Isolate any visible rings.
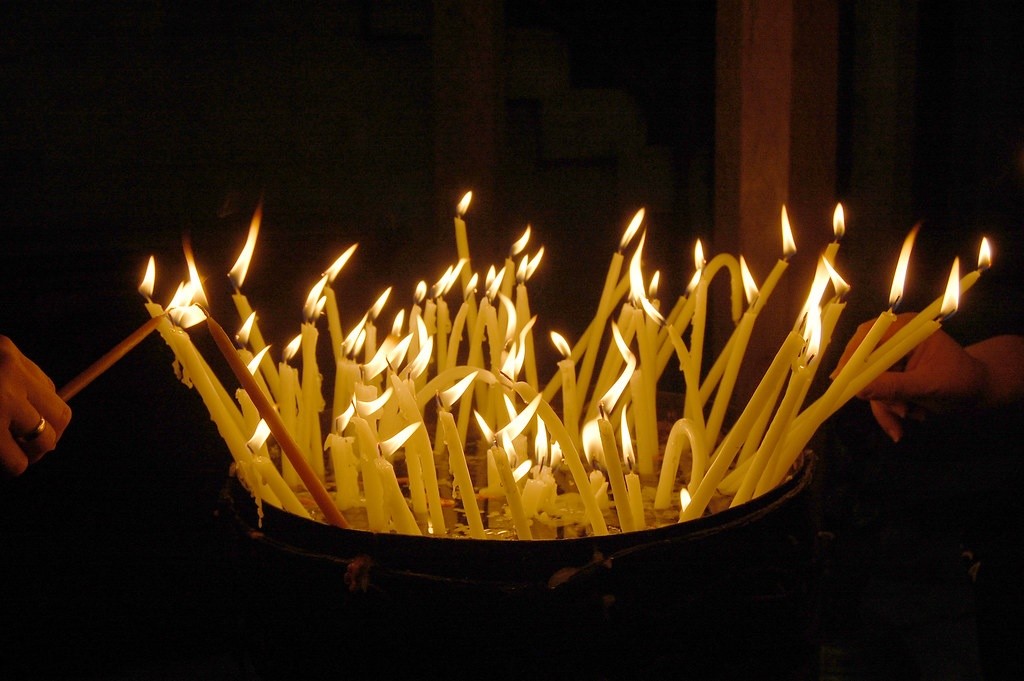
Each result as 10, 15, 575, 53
18, 415, 46, 444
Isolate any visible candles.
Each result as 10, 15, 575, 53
137, 192, 994, 542
57, 274, 210, 404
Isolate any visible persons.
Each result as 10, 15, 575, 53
0, 334, 73, 477
827, 310, 1024, 444
